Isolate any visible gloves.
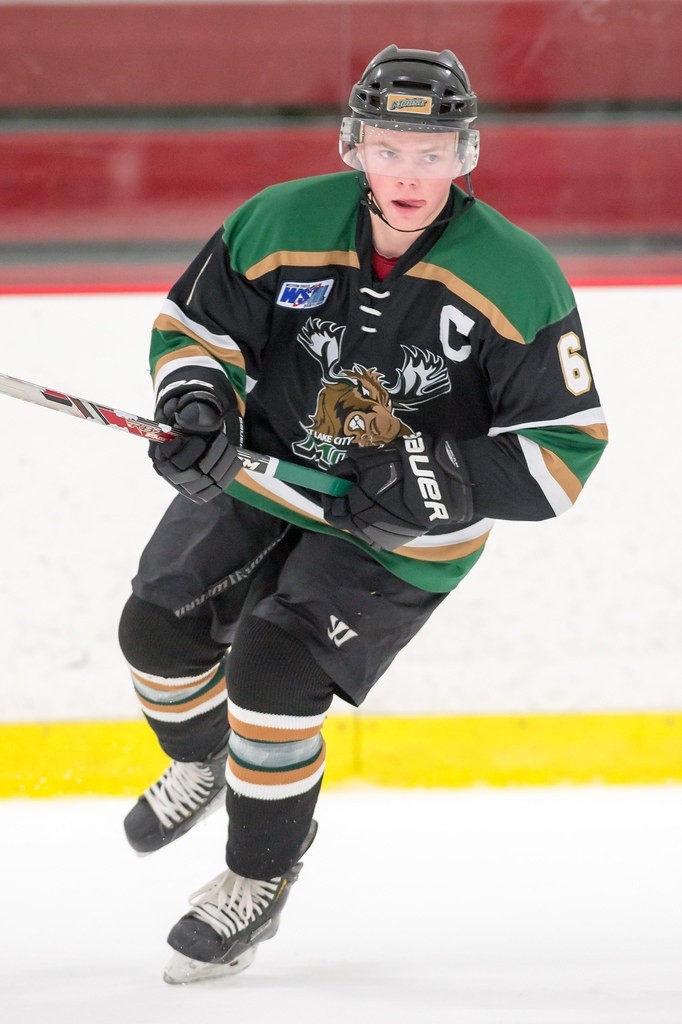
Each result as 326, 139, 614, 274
146, 389, 243, 506
319, 432, 474, 530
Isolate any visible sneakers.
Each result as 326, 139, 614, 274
123, 727, 231, 857
163, 819, 319, 985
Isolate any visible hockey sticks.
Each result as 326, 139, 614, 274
0, 369, 356, 498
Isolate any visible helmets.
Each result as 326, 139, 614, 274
348, 43, 478, 160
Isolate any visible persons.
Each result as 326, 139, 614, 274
115, 43, 611, 986
